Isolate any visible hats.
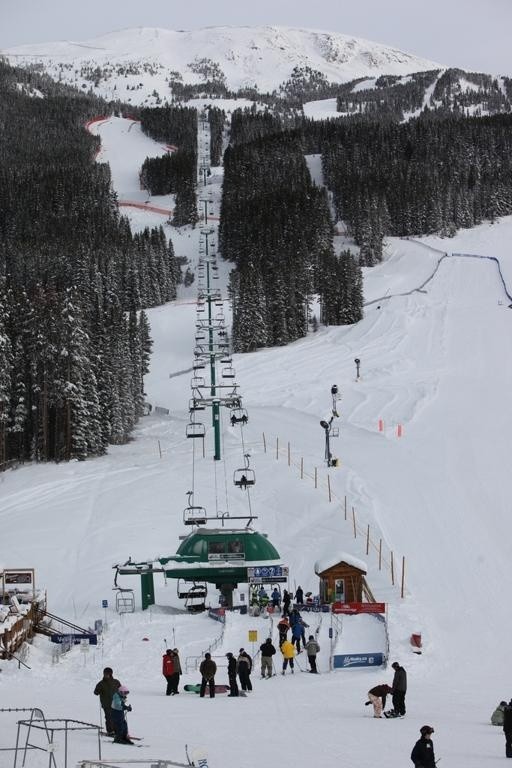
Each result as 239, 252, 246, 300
420, 726, 433, 736
118, 687, 129, 695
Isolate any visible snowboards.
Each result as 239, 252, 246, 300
384, 710, 402, 717
184, 685, 226, 693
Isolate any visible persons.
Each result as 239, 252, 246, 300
501, 697, 512, 758
367, 683, 391, 719
159, 579, 322, 700
111, 685, 136, 745
491, 699, 507, 726
231, 414, 237, 426
410, 724, 437, 768
93, 666, 123, 733
242, 413, 248, 425
389, 661, 408, 718
238, 475, 248, 489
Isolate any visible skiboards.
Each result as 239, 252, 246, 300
260, 665, 321, 680
98, 730, 151, 749
